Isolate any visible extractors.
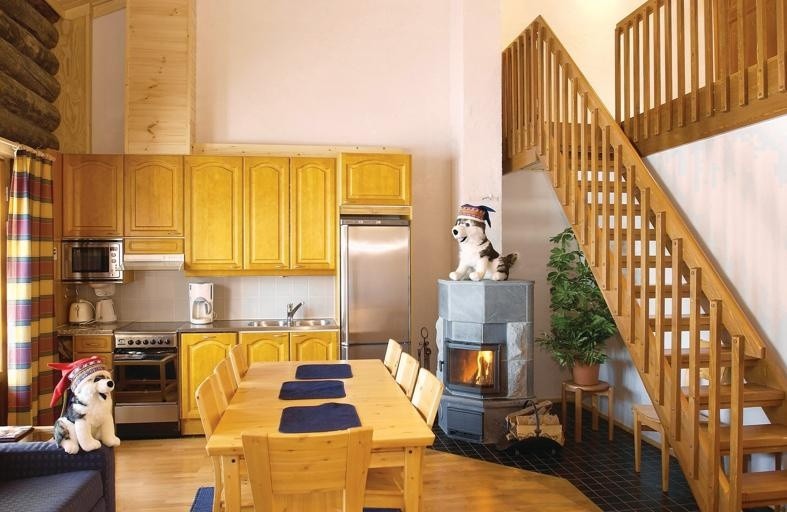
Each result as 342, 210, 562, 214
122, 254, 184, 273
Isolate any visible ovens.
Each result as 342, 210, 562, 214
112, 348, 183, 441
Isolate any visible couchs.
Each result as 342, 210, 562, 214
1, 441, 115, 512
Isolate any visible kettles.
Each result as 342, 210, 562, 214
95, 298, 117, 323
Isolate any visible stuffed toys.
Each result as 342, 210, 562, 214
47, 355, 121, 455
448, 204, 518, 281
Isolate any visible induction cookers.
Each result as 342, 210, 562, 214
112, 319, 186, 348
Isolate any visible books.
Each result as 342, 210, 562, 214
0, 425, 33, 442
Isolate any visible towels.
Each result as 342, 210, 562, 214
296, 364, 353, 380
279, 403, 362, 432
279, 381, 346, 400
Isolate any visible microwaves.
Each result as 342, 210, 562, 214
60, 239, 124, 285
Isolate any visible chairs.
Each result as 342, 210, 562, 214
394, 351, 420, 400
241, 426, 374, 512
631, 339, 730, 491
228, 342, 249, 384
195, 375, 249, 512
364, 367, 445, 511
214, 359, 235, 405
384, 338, 403, 377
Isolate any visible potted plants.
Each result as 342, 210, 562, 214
532, 227, 618, 385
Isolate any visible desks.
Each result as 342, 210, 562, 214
0, 426, 35, 443
204, 358, 435, 512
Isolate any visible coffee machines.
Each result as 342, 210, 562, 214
188, 281, 214, 325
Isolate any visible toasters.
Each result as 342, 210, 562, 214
68, 300, 94, 324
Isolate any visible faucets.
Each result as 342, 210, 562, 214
285, 300, 305, 320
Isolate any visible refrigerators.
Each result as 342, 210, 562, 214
339, 217, 411, 362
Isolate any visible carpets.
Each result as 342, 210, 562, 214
190, 487, 403, 512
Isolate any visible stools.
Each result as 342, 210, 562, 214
561, 379, 615, 443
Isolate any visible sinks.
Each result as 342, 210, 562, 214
246, 318, 331, 329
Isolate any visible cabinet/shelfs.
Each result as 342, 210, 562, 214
72, 334, 113, 372
340, 151, 412, 206
184, 156, 243, 271
62, 153, 184, 255
179, 332, 238, 435
239, 330, 341, 367
244, 156, 337, 271
